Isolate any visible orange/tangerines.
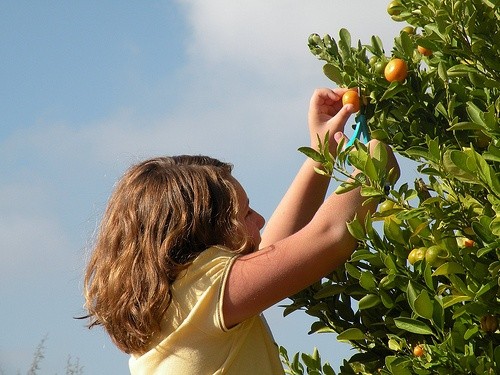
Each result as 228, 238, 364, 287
380, 198, 475, 266
414, 345, 423, 358
341, 1, 433, 114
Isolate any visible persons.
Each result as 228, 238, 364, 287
74, 86, 402, 375
